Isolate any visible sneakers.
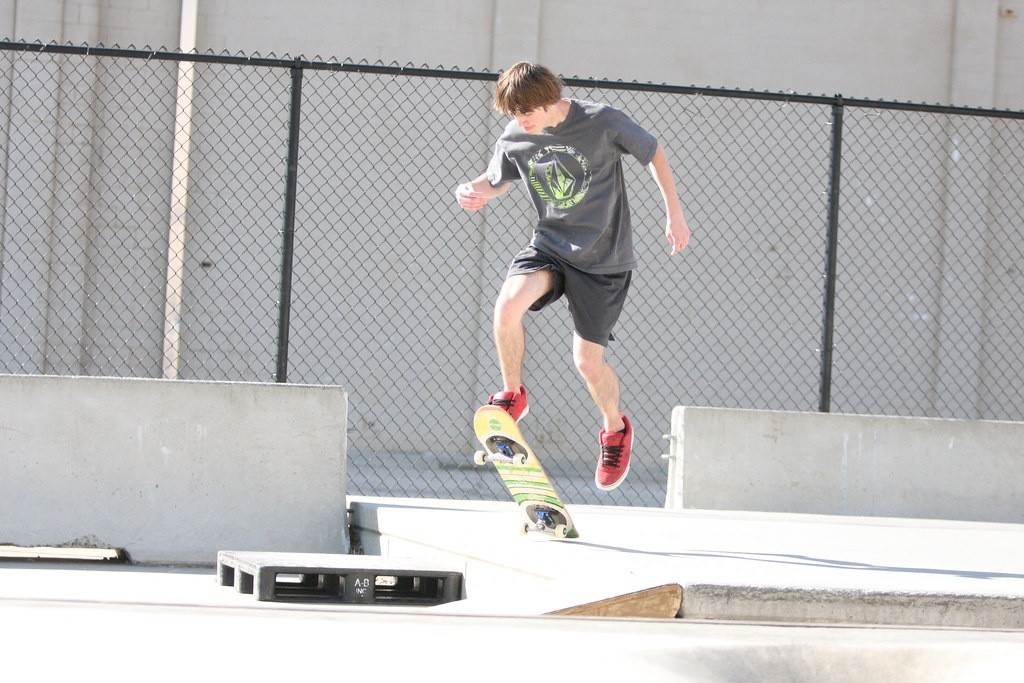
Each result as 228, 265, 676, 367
595, 412, 634, 492
487, 384, 529, 424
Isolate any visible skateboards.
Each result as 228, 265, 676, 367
473, 405, 579, 538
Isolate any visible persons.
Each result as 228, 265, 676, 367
455, 61, 689, 491
